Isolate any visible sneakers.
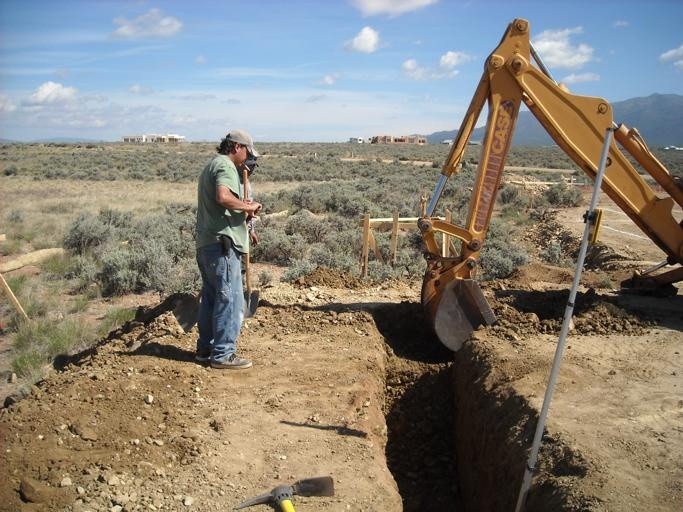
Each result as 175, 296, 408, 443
194, 354, 211, 361
210, 354, 253, 370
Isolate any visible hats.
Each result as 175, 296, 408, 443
226, 129, 261, 168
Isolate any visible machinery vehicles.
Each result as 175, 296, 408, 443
410, 20, 682, 354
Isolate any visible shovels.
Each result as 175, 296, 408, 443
242, 169, 259, 319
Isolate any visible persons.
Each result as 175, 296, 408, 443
196, 128, 262, 369
235, 147, 259, 318
